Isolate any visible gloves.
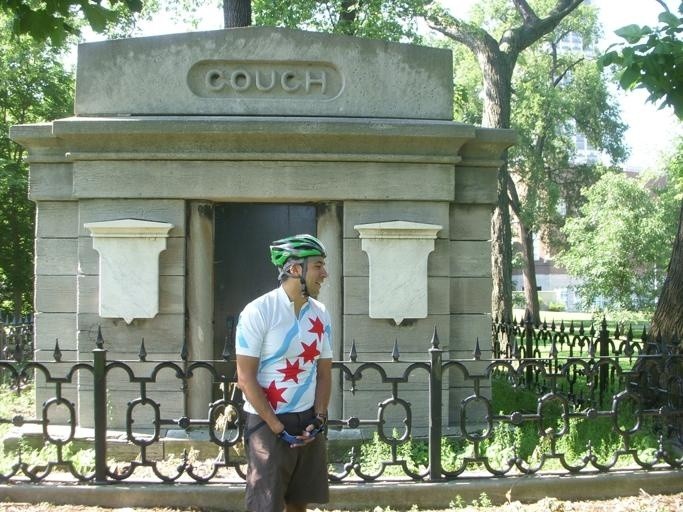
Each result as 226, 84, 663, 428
276, 418, 324, 445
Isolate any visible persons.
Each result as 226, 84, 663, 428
235, 235, 332, 511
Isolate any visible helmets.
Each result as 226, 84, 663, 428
269, 234, 326, 266
